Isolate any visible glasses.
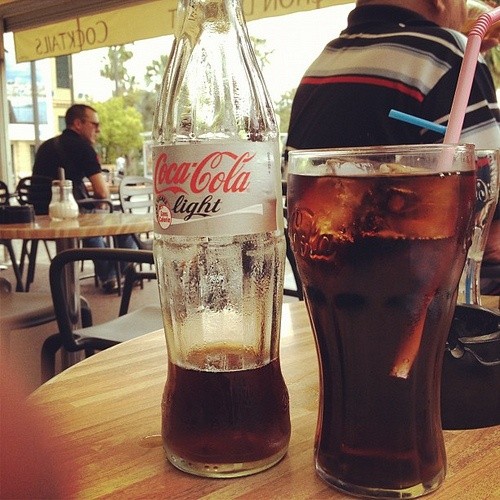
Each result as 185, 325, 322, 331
89, 121, 99, 128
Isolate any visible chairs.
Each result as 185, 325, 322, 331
0, 175, 303, 385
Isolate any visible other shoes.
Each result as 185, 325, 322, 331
101, 275, 126, 292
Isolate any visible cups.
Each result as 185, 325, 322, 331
287, 143, 474, 500
455, 148, 498, 305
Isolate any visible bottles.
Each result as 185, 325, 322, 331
48, 180, 80, 221
149, 0, 291, 479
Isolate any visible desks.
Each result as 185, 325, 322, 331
27, 296, 500, 500
0, 212, 153, 371
85, 181, 121, 211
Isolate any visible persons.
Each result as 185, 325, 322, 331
28, 104, 143, 295
283, 1, 500, 298
